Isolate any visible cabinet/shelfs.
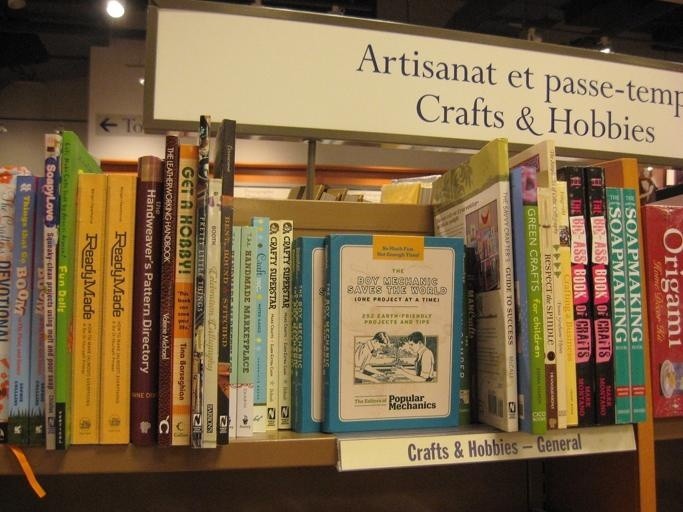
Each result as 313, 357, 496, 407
0, 157, 682, 512
